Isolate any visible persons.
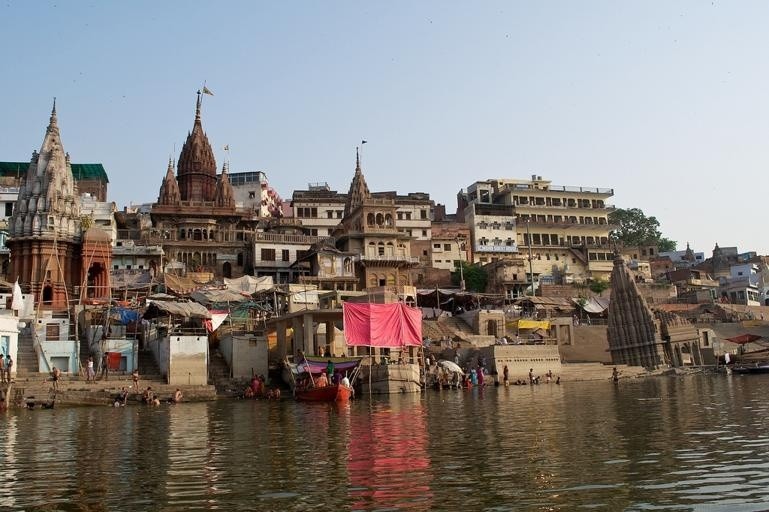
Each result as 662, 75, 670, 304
721, 295, 730, 304
5, 355, 13, 382
733, 309, 764, 321
0, 397, 5, 411
609, 367, 622, 382
52, 366, 59, 390
0, 354, 5, 382
422, 335, 560, 389
587, 313, 591, 325
453, 300, 477, 315
86, 357, 96, 381
573, 313, 579, 326
523, 306, 528, 318
294, 344, 351, 387
115, 366, 183, 406
243, 374, 281, 400
101, 351, 111, 380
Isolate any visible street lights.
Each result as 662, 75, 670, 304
523, 216, 535, 297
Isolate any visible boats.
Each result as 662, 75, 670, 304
294, 382, 352, 402
733, 363, 769, 374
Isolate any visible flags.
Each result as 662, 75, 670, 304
223, 144, 229, 150
202, 85, 214, 97
361, 140, 368, 145
